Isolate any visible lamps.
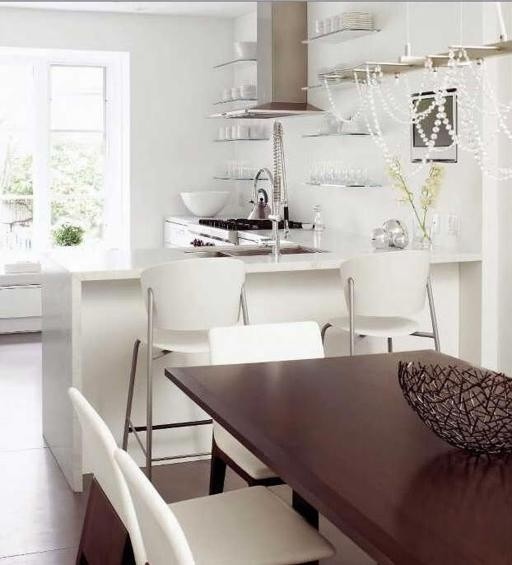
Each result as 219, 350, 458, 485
322, 0, 512, 181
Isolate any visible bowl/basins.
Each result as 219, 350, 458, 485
301, 223, 314, 231
232, 86, 256, 100
235, 41, 258, 59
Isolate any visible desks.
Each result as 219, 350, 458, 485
164, 346, 511, 565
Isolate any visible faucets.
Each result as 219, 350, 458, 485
250, 166, 283, 264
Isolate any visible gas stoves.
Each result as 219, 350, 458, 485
187, 219, 315, 249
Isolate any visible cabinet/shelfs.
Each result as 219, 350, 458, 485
211, 58, 270, 183
302, 28, 390, 191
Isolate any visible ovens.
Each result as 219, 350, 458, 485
410, 92, 458, 163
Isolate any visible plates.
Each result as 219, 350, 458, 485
340, 11, 374, 30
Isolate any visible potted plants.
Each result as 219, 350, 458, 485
50, 221, 86, 256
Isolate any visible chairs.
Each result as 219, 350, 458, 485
209, 320, 326, 533
67, 385, 338, 565
124, 257, 248, 482
321, 252, 439, 356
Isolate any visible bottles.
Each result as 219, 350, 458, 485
311, 203, 321, 232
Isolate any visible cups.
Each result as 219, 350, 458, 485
316, 16, 341, 35
218, 126, 249, 139
222, 161, 260, 180
309, 160, 368, 185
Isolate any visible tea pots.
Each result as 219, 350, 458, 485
248, 187, 272, 220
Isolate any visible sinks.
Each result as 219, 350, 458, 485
227, 243, 323, 258
185, 247, 233, 258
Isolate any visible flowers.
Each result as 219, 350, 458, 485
388, 157, 445, 240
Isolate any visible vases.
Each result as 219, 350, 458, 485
414, 212, 437, 252
